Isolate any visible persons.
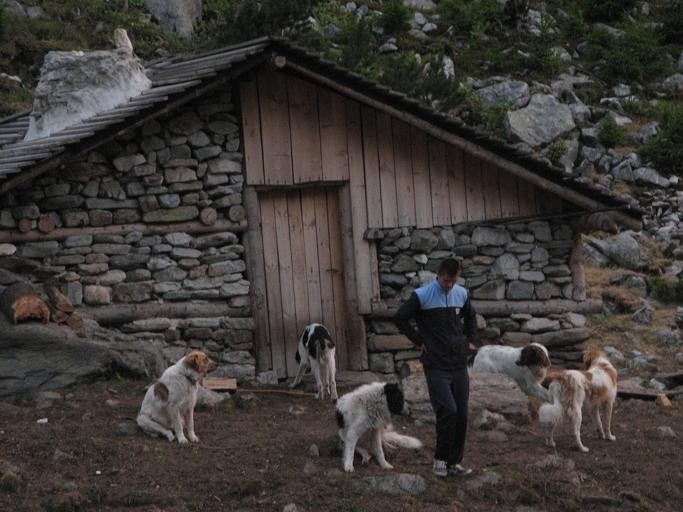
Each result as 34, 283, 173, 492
393, 258, 479, 477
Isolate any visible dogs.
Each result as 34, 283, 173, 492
137, 350, 220, 446
461, 342, 553, 406
290, 322, 337, 403
537, 350, 618, 453
335, 381, 422, 473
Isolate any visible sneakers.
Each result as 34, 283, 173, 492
432, 457, 473, 477
